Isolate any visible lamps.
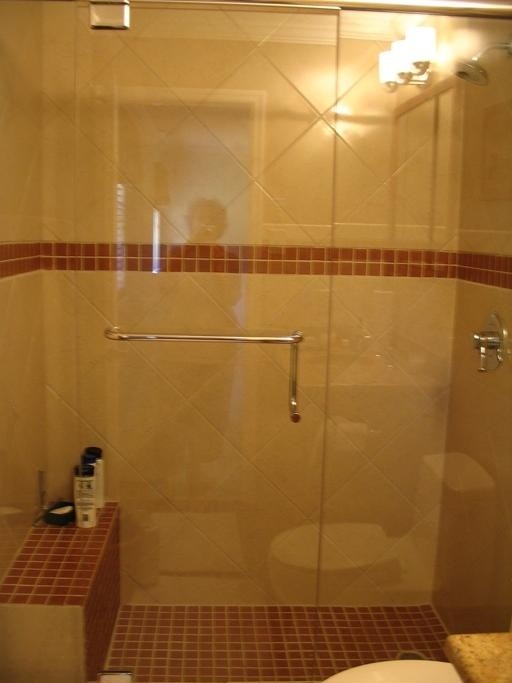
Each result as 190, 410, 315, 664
379, 25, 437, 93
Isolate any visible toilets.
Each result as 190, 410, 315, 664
321, 659, 462, 682
269, 451, 496, 610
